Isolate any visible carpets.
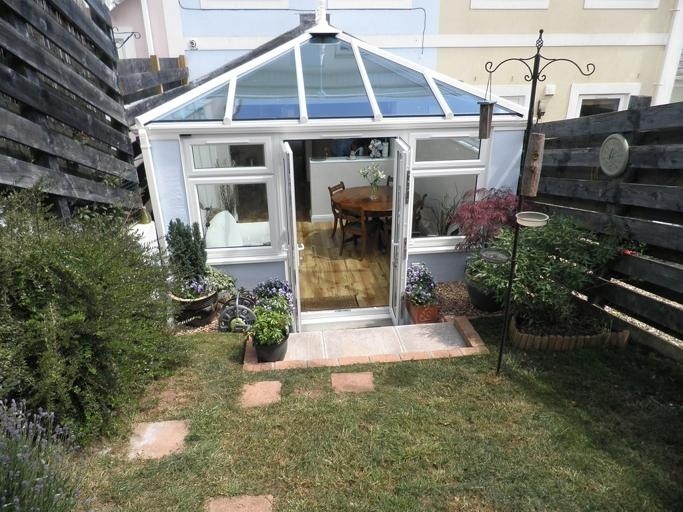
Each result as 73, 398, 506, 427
300, 293, 360, 311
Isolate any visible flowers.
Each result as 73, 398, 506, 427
170, 272, 218, 299
402, 263, 444, 308
359, 164, 387, 195
251, 278, 296, 326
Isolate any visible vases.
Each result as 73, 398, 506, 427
168, 291, 219, 327
367, 183, 382, 201
406, 300, 443, 324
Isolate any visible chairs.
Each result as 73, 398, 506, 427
328, 176, 427, 261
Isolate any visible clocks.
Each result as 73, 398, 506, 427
598, 133, 629, 178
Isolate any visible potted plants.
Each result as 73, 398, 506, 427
240, 305, 294, 362
